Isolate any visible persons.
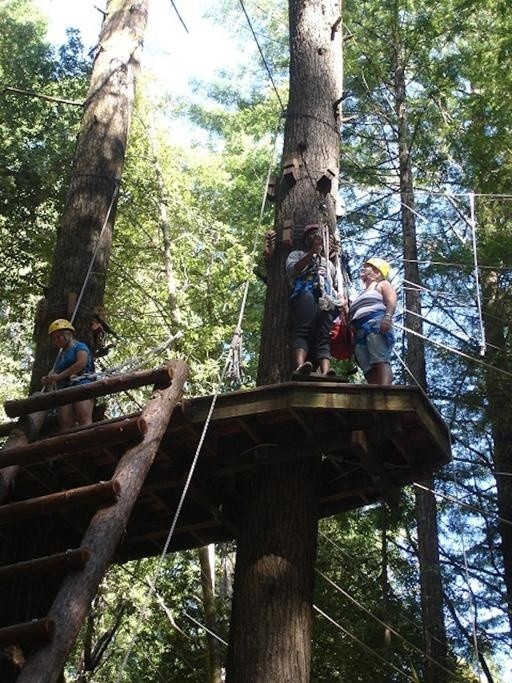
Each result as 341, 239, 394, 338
286, 223, 339, 376
39, 317, 96, 430
346, 257, 398, 383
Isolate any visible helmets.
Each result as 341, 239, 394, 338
48, 319, 75, 335
367, 258, 389, 279
303, 224, 319, 233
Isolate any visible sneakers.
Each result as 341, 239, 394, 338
296, 361, 313, 372
326, 368, 337, 376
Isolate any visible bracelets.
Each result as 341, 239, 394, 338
383, 313, 393, 320
